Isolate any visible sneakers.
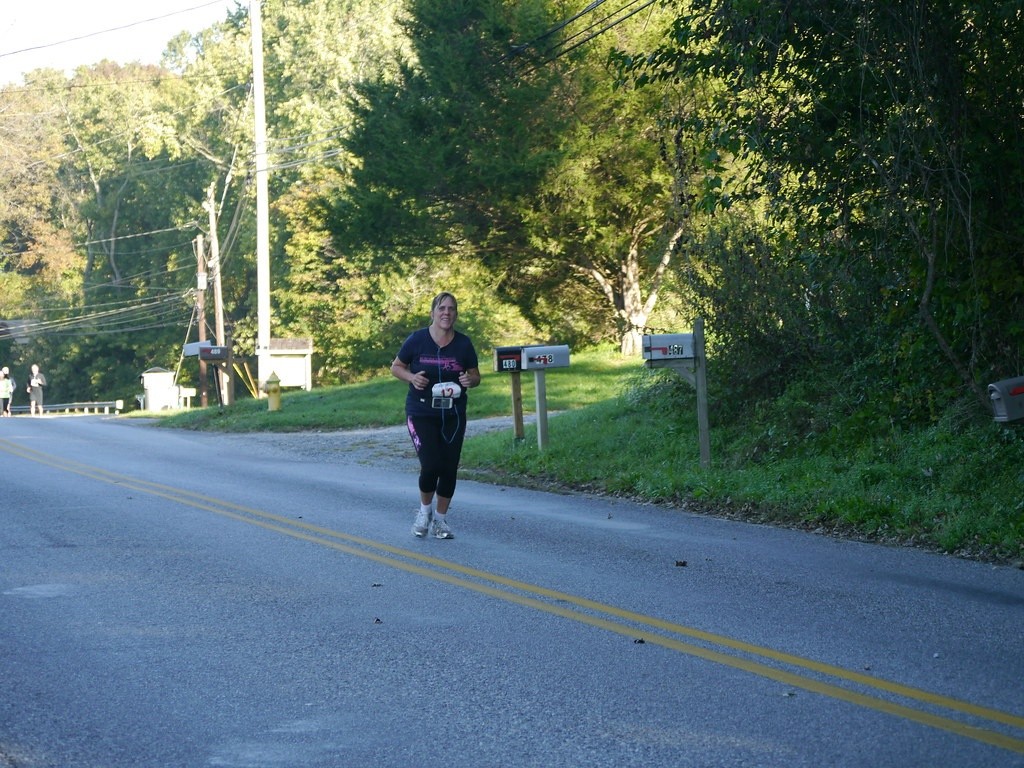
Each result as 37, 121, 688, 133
430, 517, 454, 539
411, 510, 433, 538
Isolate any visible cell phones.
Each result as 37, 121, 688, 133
432, 398, 453, 409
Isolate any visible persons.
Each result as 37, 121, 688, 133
25, 363, 47, 417
0, 365, 17, 417
390, 292, 481, 542
